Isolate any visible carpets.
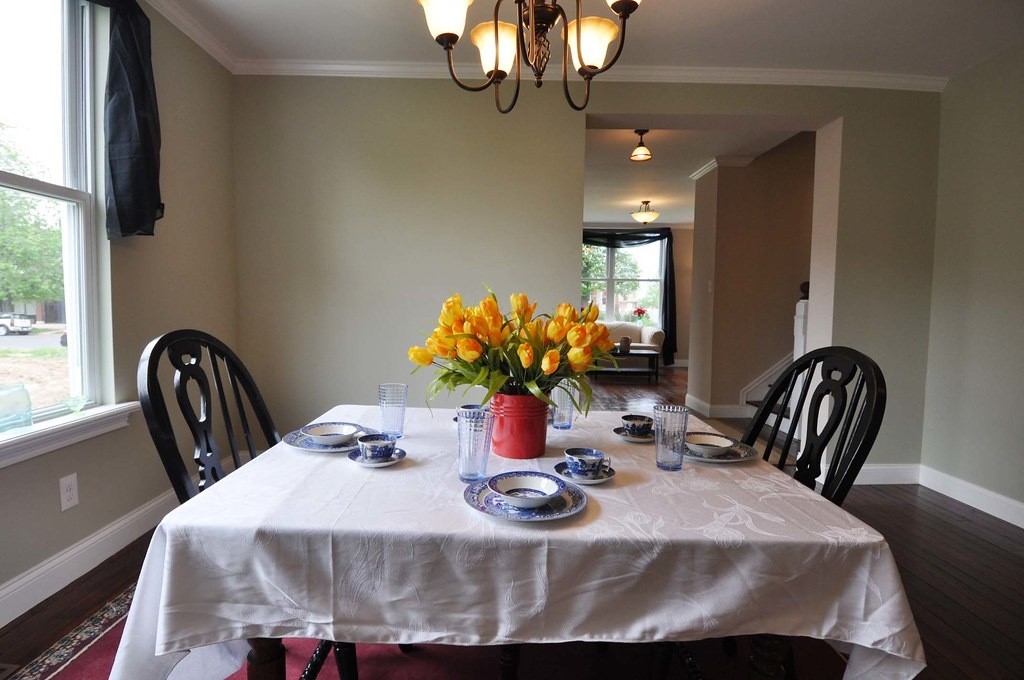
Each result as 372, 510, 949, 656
6, 578, 848, 680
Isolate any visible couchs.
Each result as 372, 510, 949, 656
594, 320, 665, 368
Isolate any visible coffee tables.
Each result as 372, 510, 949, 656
592, 350, 661, 385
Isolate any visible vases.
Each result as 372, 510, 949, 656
638, 319, 642, 325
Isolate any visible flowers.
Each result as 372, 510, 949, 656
632, 307, 647, 319
405, 284, 618, 417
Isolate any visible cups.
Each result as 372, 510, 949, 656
564, 447, 611, 480
551, 378, 576, 430
653, 405, 689, 472
621, 415, 653, 438
357, 434, 397, 463
377, 382, 408, 438
457, 409, 494, 484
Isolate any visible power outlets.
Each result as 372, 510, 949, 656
59, 473, 79, 512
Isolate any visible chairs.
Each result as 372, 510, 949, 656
137, 329, 414, 680
600, 346, 886, 680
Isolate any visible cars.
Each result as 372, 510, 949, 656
60, 328, 67, 346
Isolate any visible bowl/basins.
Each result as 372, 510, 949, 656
300, 421, 363, 445
684, 431, 739, 457
460, 404, 489, 419
486, 471, 566, 509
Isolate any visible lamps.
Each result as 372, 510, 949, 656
630, 201, 660, 226
630, 129, 652, 161
416, 0, 642, 114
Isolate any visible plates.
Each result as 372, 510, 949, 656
683, 440, 758, 464
553, 461, 616, 485
612, 426, 656, 443
348, 448, 407, 468
282, 426, 380, 454
463, 477, 588, 522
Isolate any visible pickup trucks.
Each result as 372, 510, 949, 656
0, 318, 31, 337
